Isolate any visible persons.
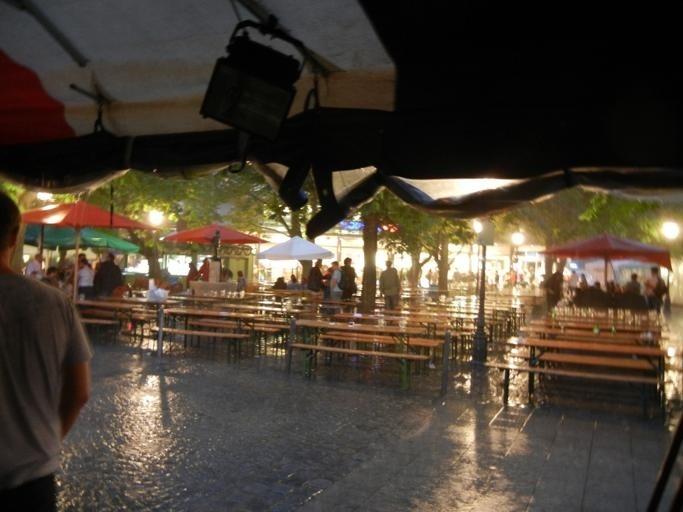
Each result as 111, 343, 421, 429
0, 191, 93, 512
379, 261, 401, 309
494, 269, 529, 287
186, 258, 209, 290
23, 252, 123, 301
274, 257, 358, 301
238, 271, 246, 291
546, 263, 664, 316
427, 269, 475, 286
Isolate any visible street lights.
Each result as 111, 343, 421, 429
511, 230, 524, 289
148, 209, 165, 275
662, 220, 679, 311
34, 192, 54, 259
471, 220, 496, 361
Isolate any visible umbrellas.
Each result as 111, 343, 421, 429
533, 231, 672, 287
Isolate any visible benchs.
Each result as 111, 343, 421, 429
80, 291, 668, 420
